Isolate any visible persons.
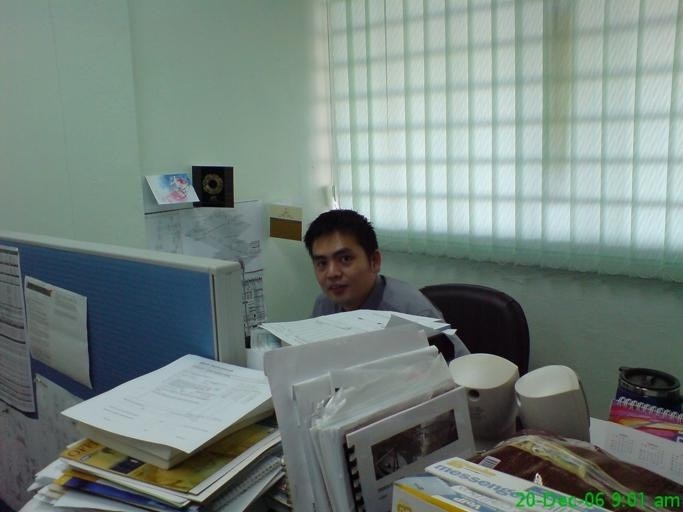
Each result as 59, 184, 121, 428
303, 210, 470, 363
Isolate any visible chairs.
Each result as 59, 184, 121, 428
418, 283, 530, 395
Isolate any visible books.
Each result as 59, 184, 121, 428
25, 324, 476, 512
609, 396, 683, 423
